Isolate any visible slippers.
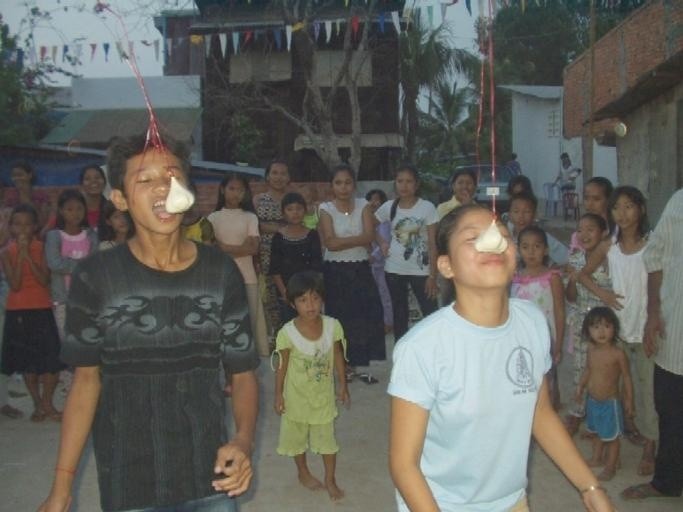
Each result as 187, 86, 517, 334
620, 483, 680, 503
31, 407, 63, 423
358, 372, 379, 385
345, 370, 354, 383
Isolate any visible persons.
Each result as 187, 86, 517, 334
497, 152, 683, 501
34, 132, 261, 512
384, 204, 622, 512
2, 163, 133, 421
268, 271, 352, 499
176, 159, 479, 385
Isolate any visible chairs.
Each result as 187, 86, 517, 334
562, 193, 580, 221
544, 182, 562, 216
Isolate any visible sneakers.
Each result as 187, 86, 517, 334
0, 405, 23, 419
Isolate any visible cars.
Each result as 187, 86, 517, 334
449, 164, 518, 218
420, 171, 448, 187
